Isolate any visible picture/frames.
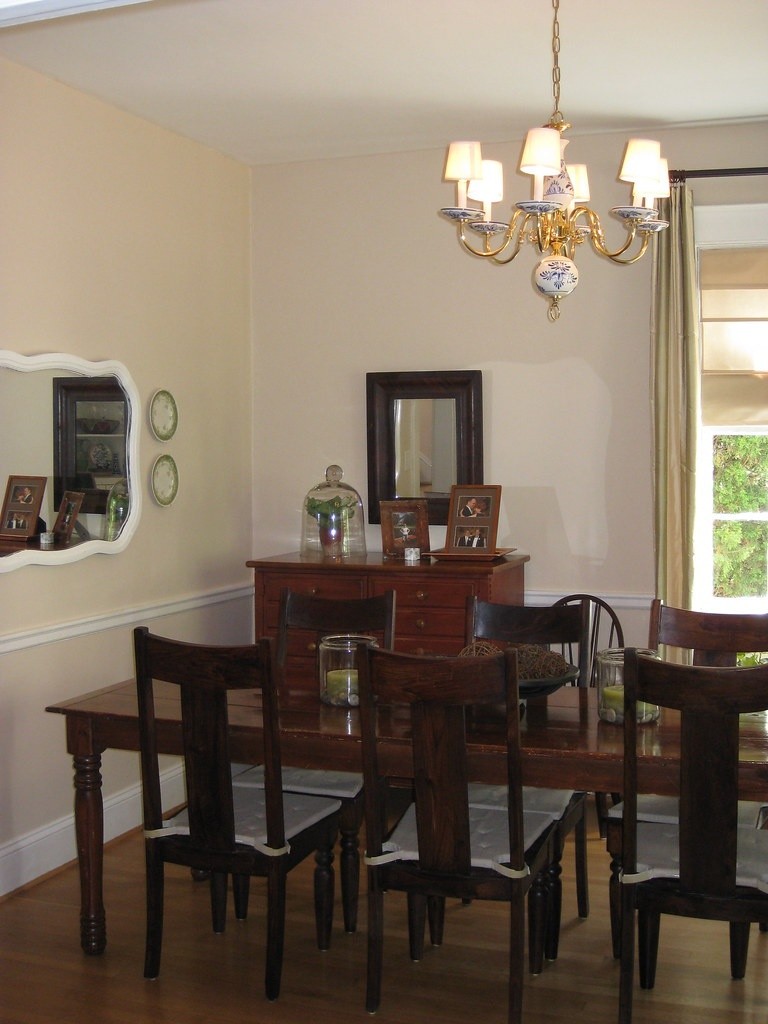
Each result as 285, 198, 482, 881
379, 498, 431, 561
51, 491, 85, 545
1, 475, 47, 537
444, 485, 502, 554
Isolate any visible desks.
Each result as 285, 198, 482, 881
46, 676, 768, 957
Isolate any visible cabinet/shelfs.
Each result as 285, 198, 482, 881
52, 374, 128, 515
246, 550, 531, 692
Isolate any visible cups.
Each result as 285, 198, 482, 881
40, 532, 54, 543
404, 548, 420, 561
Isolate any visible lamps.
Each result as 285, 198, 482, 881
440, 0, 671, 322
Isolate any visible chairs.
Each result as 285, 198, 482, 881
546, 596, 626, 840
354, 642, 558, 1024
426, 594, 593, 964
616, 649, 768, 1024
604, 601, 768, 962
232, 588, 396, 933
132, 625, 344, 1004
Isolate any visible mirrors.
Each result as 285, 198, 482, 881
1, 349, 145, 572
366, 367, 483, 527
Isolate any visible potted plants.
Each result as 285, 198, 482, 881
304, 496, 359, 556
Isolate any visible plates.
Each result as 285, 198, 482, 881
151, 454, 179, 508
149, 389, 178, 441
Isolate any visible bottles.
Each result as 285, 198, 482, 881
319, 634, 382, 708
597, 648, 662, 725
